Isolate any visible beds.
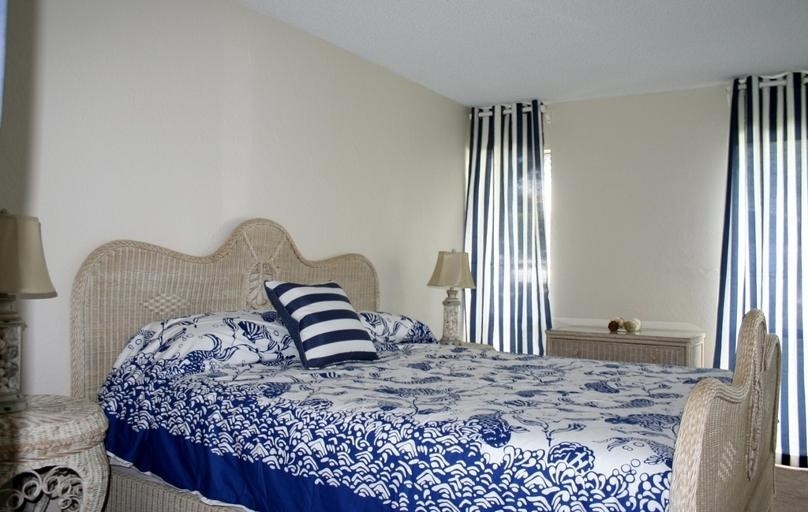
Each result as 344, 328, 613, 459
69, 216, 783, 512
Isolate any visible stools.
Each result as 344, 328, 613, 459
0, 391, 111, 512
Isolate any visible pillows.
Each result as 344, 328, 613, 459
265, 274, 382, 370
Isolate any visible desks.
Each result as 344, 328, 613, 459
544, 325, 707, 369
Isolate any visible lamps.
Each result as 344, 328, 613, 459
425, 247, 479, 348
1, 204, 58, 414
